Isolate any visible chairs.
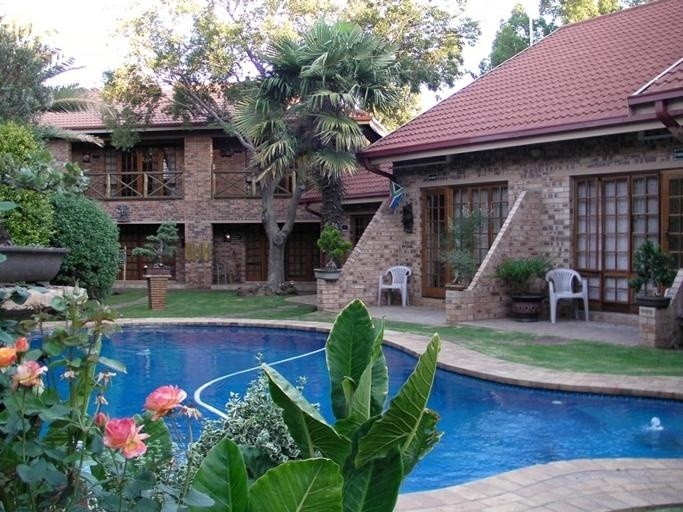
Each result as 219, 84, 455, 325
544, 268, 589, 323
377, 266, 412, 307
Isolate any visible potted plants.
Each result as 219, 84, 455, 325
627, 241, 679, 309
437, 205, 489, 291
0, 118, 71, 287
485, 256, 552, 323
312, 222, 353, 280
131, 219, 179, 276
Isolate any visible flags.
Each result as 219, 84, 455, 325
384, 179, 406, 212
